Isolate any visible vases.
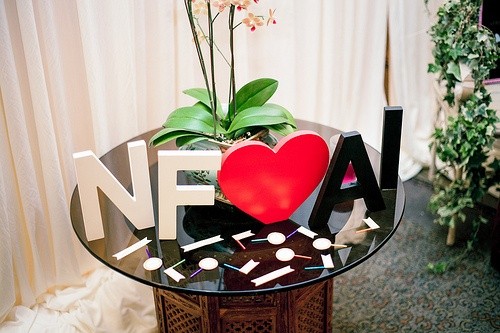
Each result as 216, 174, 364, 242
179, 126, 273, 206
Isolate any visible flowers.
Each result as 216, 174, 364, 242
150, 0, 299, 153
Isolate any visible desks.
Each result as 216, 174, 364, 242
70, 110, 406, 333
